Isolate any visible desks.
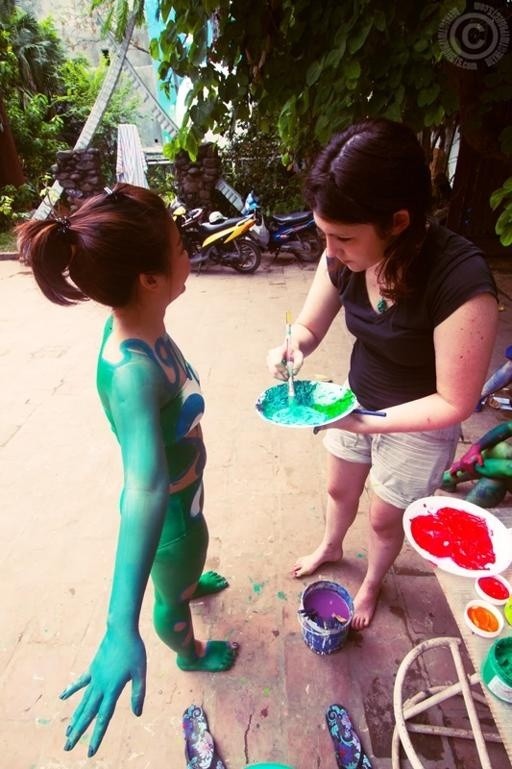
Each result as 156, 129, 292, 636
389, 523, 512, 769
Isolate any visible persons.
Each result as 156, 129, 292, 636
16, 184, 242, 754
474, 345, 512, 413
268, 116, 501, 630
439, 420, 512, 509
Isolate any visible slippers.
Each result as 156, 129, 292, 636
182, 704, 225, 768
325, 702, 373, 768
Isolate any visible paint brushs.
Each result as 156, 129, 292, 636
284, 316, 297, 405
297, 609, 348, 630
352, 408, 387, 417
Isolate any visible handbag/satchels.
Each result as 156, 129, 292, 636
249, 214, 271, 245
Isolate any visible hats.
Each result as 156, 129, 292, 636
208, 210, 229, 223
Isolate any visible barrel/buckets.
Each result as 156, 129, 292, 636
297, 579, 355, 657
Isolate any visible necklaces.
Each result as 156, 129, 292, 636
367, 270, 398, 316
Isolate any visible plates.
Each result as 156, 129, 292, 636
474, 572, 512, 604
253, 379, 358, 427
402, 496, 512, 577
463, 599, 504, 638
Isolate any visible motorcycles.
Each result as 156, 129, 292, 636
172, 206, 264, 275
235, 184, 324, 263
